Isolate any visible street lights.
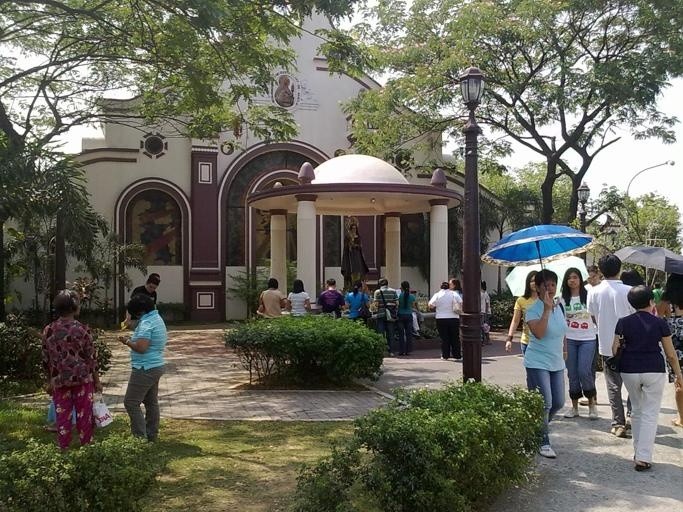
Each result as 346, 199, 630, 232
577, 181, 589, 269
625, 159, 675, 249
458, 66, 486, 386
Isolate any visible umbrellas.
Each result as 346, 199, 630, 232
481, 220, 596, 307
614, 246, 683, 274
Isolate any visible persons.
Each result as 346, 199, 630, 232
505, 253, 683, 472
341, 223, 369, 280
123, 273, 160, 328
374, 278, 399, 357
118, 296, 166, 442
345, 280, 370, 324
398, 278, 493, 361
41, 290, 102, 453
287, 279, 311, 316
316, 278, 345, 319
259, 278, 287, 359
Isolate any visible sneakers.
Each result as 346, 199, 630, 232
540, 400, 683, 472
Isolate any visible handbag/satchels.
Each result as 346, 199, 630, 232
453, 299, 462, 314
385, 302, 398, 321
93, 391, 113, 427
48, 400, 76, 425
605, 334, 626, 371
